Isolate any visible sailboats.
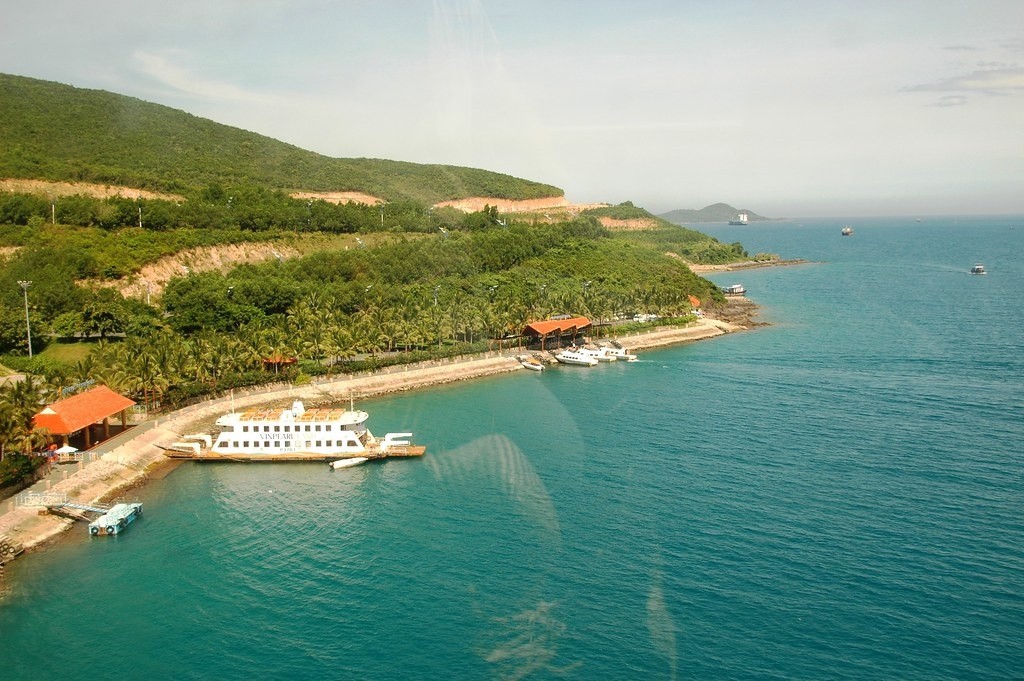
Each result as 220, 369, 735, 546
727, 214, 749, 225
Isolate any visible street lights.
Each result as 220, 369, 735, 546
17, 280, 33, 358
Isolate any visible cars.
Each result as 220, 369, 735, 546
633, 314, 659, 323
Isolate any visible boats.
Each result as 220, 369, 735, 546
329, 457, 369, 470
724, 284, 747, 296
522, 362, 545, 371
599, 347, 637, 362
163, 388, 426, 470
577, 349, 616, 362
841, 225, 853, 236
555, 351, 598, 367
971, 266, 987, 275
